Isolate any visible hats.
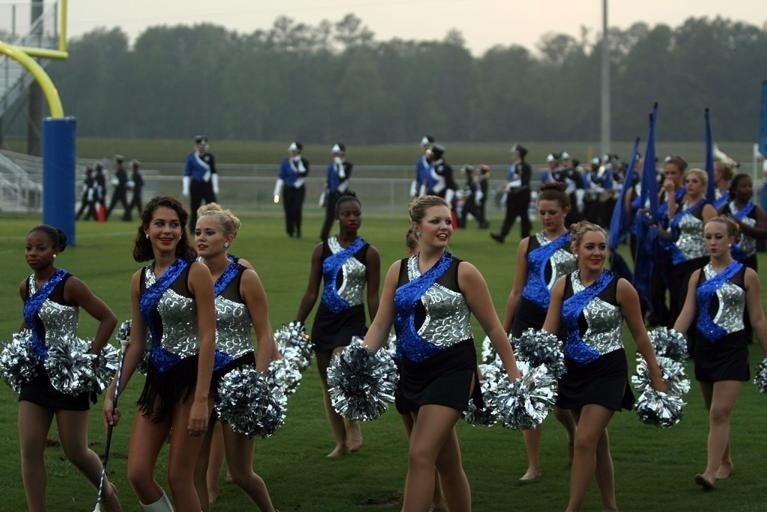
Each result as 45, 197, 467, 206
430, 143, 443, 157
196, 136, 208, 143
334, 144, 345, 156
422, 137, 435, 146
514, 146, 528, 155
289, 143, 298, 153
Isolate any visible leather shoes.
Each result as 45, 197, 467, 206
490, 233, 503, 244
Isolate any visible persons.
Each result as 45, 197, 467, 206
490, 143, 532, 243
74, 165, 97, 220
477, 164, 488, 217
541, 151, 627, 230
410, 135, 435, 197
206, 419, 233, 503
103, 195, 215, 511
182, 136, 219, 235
361, 193, 521, 512
637, 154, 688, 330
397, 226, 446, 512
17, 225, 121, 512
717, 173, 767, 345
297, 191, 380, 458
106, 155, 132, 221
672, 213, 767, 490
652, 155, 665, 203
457, 164, 489, 228
192, 202, 277, 512
632, 153, 641, 185
637, 168, 718, 360
419, 143, 456, 211
120, 158, 144, 222
711, 160, 739, 211
503, 182, 579, 482
83, 162, 108, 222
539, 221, 669, 512
319, 143, 353, 243
625, 157, 646, 326
273, 142, 309, 238
